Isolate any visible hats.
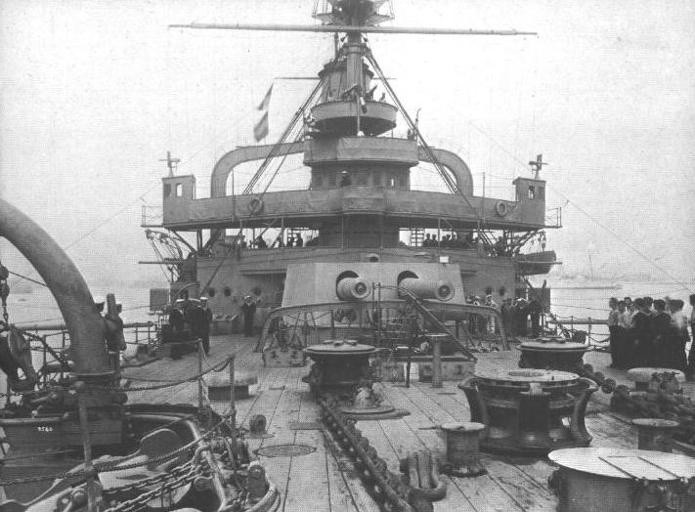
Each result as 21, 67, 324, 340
200, 296, 208, 301
177, 298, 185, 303
96, 301, 105, 305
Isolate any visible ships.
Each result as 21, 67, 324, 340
1, 2, 694, 512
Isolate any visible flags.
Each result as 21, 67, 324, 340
254, 113, 269, 142
258, 84, 271, 111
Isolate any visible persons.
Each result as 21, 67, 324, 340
105, 304, 127, 352
169, 296, 213, 356
243, 296, 256, 337
242, 233, 303, 250
467, 295, 542, 338
95, 301, 105, 316
608, 294, 695, 375
423, 232, 503, 255
